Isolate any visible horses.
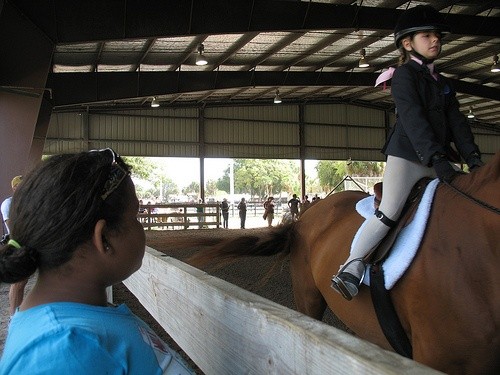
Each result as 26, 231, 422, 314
184, 149, 500, 375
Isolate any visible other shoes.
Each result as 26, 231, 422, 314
330, 272, 361, 297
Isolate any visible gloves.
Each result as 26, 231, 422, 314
431, 157, 455, 184
468, 159, 483, 173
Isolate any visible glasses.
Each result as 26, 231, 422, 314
88, 147, 120, 225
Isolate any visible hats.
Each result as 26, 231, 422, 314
12, 175, 25, 187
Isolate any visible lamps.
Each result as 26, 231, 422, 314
468, 105, 475, 118
491, 54, 500, 73
274, 89, 282, 103
195, 43, 209, 65
359, 47, 370, 67
151, 97, 160, 107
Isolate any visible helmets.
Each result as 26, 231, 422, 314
394, 5, 445, 48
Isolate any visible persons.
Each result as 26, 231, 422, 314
1, 175, 30, 327
0, 144, 199, 375
330, 5, 485, 300
139, 194, 322, 229
